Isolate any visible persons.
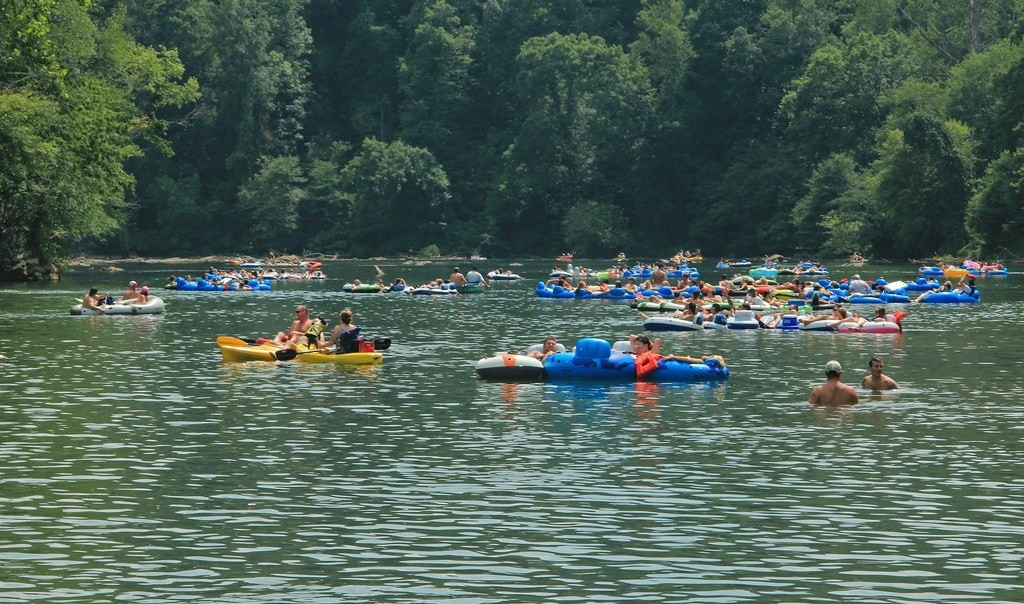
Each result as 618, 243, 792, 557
629, 334, 660, 357
269, 305, 357, 360
351, 265, 513, 289
862, 357, 899, 389
530, 337, 556, 361
168, 254, 321, 290
546, 249, 1004, 330
83, 288, 109, 312
808, 361, 858, 404
663, 354, 725, 367
118, 281, 148, 305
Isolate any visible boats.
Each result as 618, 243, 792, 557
217, 336, 383, 364
474, 343, 565, 371
535, 255, 1008, 332
164, 270, 327, 291
542, 337, 729, 381
454, 282, 483, 292
471, 256, 487, 260
487, 271, 518, 279
413, 285, 456, 295
225, 260, 243, 263
70, 294, 164, 316
383, 286, 414, 293
343, 283, 381, 292
240, 260, 322, 268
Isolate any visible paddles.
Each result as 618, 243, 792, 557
375, 339, 391, 350
276, 349, 318, 361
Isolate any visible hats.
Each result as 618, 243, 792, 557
129, 281, 138, 287
141, 286, 149, 293
826, 360, 842, 374
454, 267, 459, 271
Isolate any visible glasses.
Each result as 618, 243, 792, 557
296, 310, 305, 313
634, 344, 643, 350
